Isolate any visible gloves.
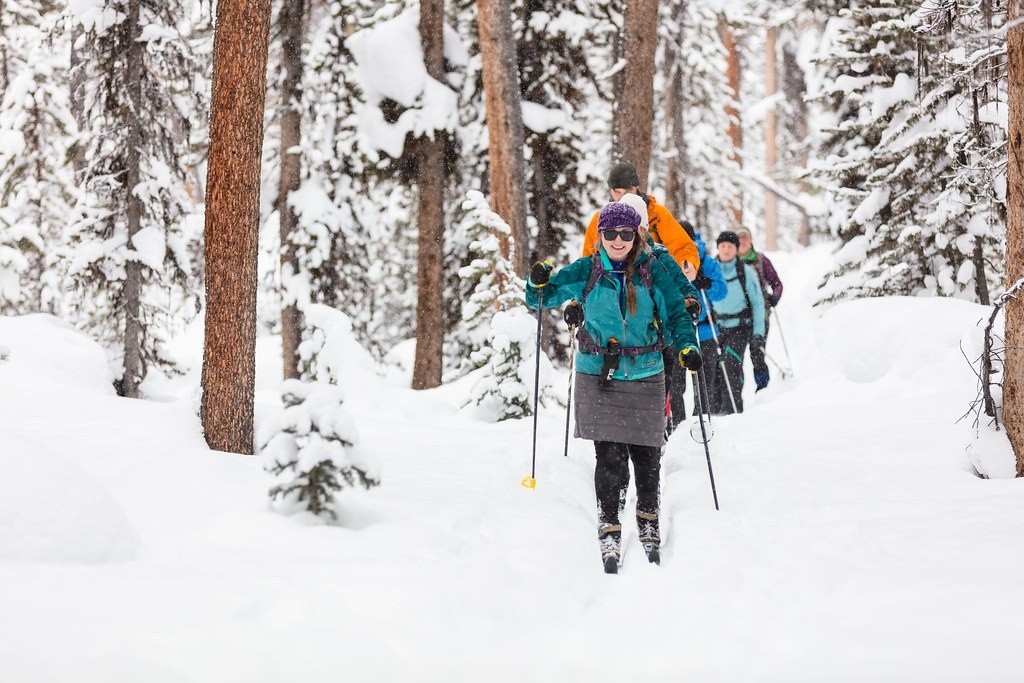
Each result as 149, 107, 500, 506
686, 302, 700, 321
682, 350, 702, 371
563, 304, 584, 327
767, 295, 779, 307
752, 335, 765, 348
693, 277, 711, 289
529, 262, 552, 286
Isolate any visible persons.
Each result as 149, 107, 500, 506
526, 202, 702, 566
580, 162, 784, 515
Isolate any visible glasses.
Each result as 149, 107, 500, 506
602, 229, 635, 241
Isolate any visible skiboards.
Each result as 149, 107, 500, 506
604, 529, 663, 576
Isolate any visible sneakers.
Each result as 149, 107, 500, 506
598, 523, 620, 562
637, 514, 661, 548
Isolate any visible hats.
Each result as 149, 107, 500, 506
598, 202, 641, 233
608, 162, 639, 188
717, 231, 740, 248
619, 192, 649, 231
679, 221, 696, 241
